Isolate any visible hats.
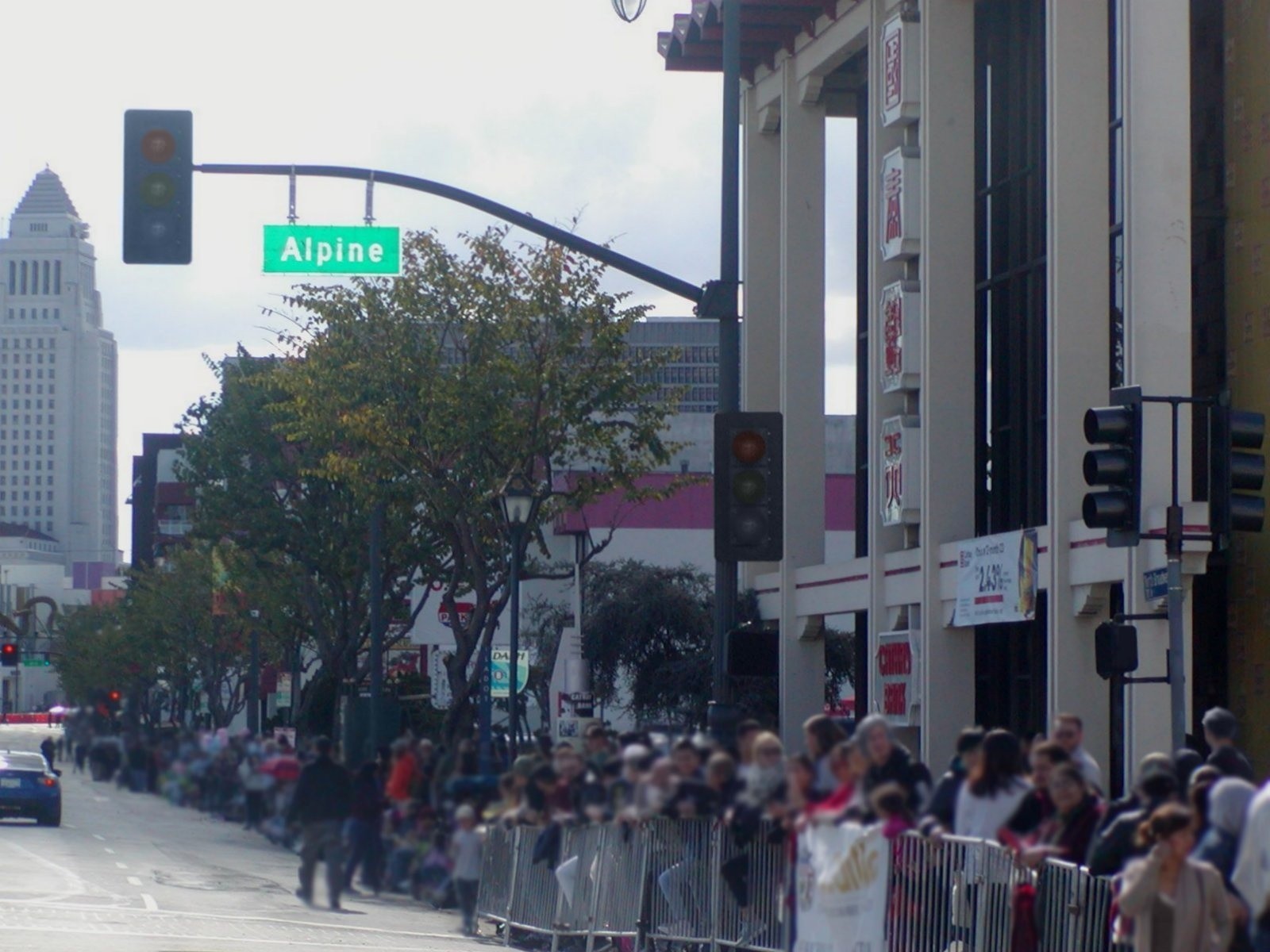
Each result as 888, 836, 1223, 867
1204, 773, 1256, 836
455, 804, 472, 818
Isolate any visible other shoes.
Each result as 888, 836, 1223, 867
552, 918, 569, 932
296, 887, 312, 905
590, 933, 613, 952
326, 902, 341, 911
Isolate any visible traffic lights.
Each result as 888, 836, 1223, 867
1, 642, 19, 665
726, 405, 788, 565
117, 105, 197, 265
1200, 400, 1268, 535
1076, 381, 1145, 554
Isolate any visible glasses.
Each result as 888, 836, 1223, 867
759, 747, 784, 757
1052, 727, 1080, 739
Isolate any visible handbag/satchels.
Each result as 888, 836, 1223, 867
1003, 848, 1038, 952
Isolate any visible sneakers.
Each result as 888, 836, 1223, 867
660, 918, 697, 938
729, 917, 768, 948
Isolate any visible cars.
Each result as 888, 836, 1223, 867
1, 749, 64, 826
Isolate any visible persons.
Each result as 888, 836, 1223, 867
32, 669, 1270, 952
